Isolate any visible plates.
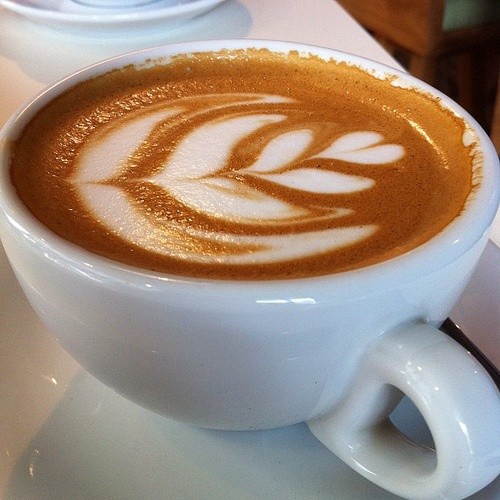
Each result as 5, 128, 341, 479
0, 0, 229, 23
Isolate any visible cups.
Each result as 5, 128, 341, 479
0, 39, 500, 500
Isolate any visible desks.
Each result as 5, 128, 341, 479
1, 0, 499, 500
